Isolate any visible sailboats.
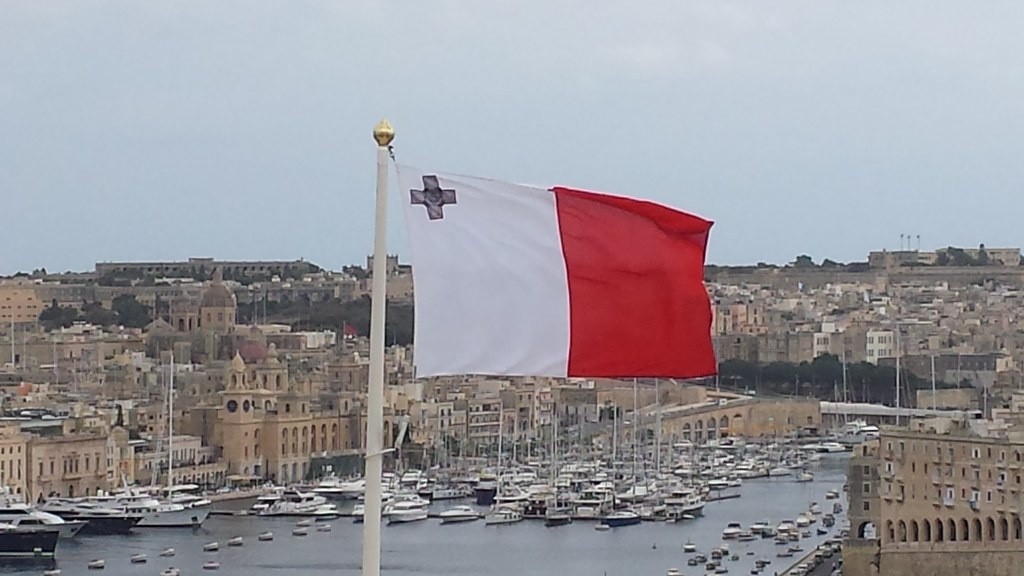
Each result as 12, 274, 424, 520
379, 374, 882, 575
130, 349, 213, 527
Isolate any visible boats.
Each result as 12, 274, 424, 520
381, 498, 431, 523
0, 528, 60, 558
438, 505, 479, 524
44, 470, 366, 576
0, 507, 88, 539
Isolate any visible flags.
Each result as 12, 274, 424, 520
344, 323, 358, 335
396, 164, 719, 379
